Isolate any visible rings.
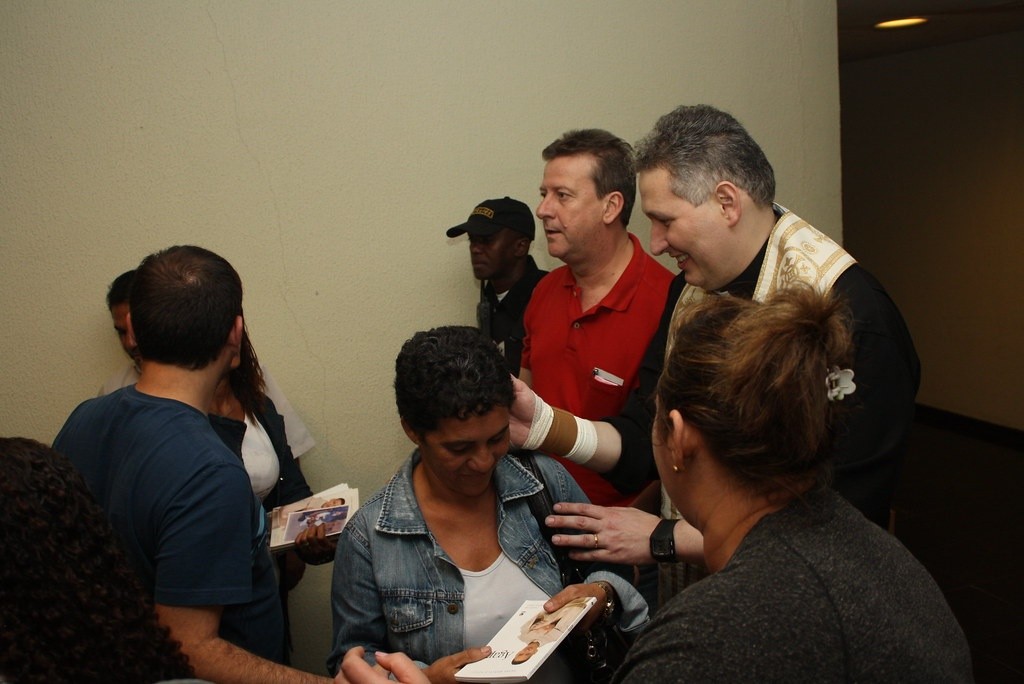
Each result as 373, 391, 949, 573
594, 534, 598, 548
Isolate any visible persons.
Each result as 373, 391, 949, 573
281, 496, 345, 520
325, 317, 651, 684
506, 104, 922, 609
47, 246, 335, 684
512, 600, 587, 665
0, 437, 193, 684
332, 293, 971, 684
109, 270, 340, 588
447, 197, 547, 378
517, 128, 675, 515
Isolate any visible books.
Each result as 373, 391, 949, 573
454, 596, 597, 684
268, 483, 360, 555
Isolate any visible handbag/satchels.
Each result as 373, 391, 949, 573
519, 450, 633, 684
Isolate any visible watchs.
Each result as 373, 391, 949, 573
650, 518, 682, 563
595, 582, 614, 618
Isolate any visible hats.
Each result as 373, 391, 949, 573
446, 195, 536, 241
